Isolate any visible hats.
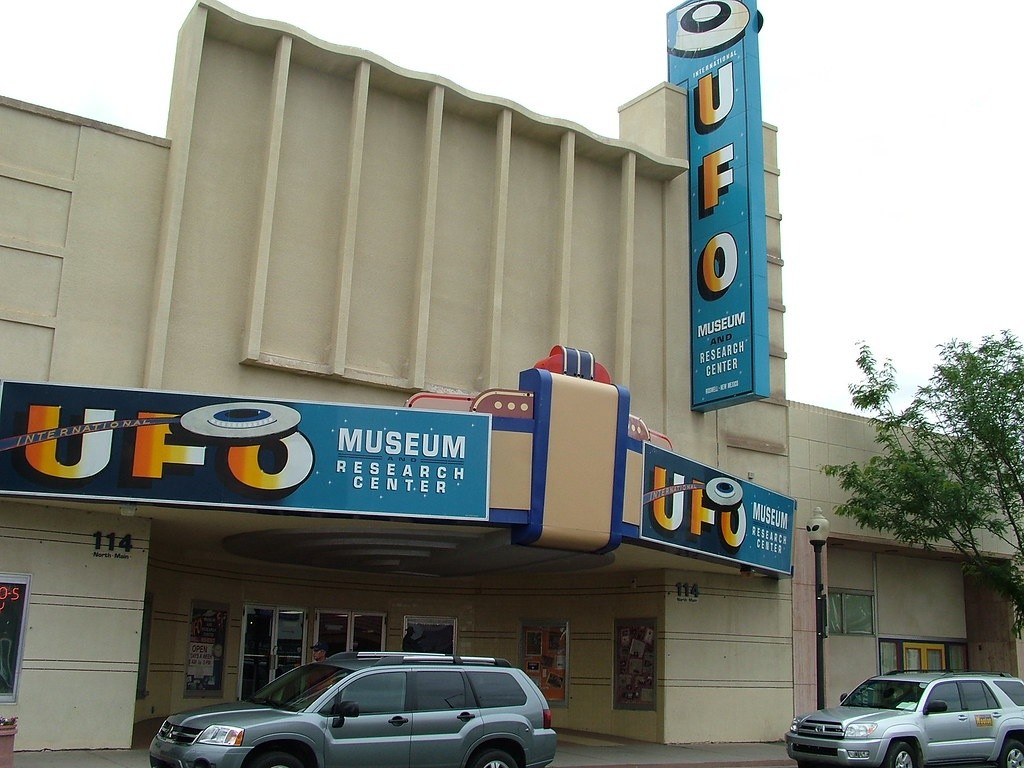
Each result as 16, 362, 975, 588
310, 641, 328, 650
405, 626, 416, 634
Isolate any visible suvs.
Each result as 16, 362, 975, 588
784, 669, 1024, 768
149, 651, 558, 768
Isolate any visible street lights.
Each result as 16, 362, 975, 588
804, 505, 829, 709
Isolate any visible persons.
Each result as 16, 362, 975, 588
305, 641, 331, 698
